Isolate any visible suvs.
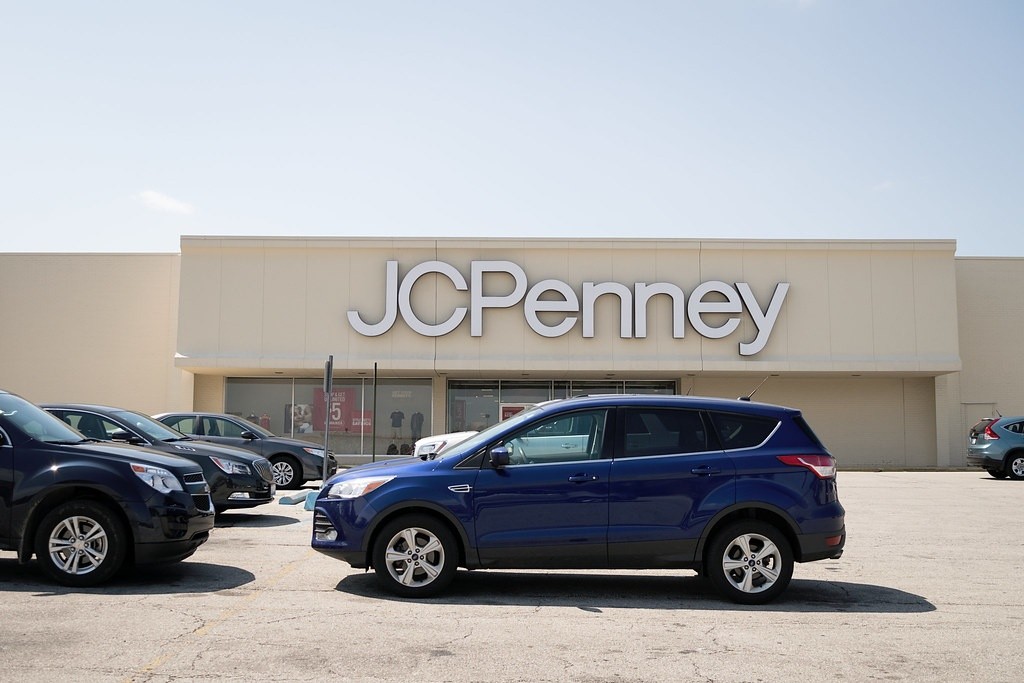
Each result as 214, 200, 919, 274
967, 415, 1024, 482
0, 386, 214, 590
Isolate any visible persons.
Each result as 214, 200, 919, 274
246, 411, 260, 426
258, 412, 272, 431
411, 411, 424, 450
389, 409, 405, 440
285, 403, 304, 434
302, 404, 314, 434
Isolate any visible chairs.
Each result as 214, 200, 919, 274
77, 414, 106, 440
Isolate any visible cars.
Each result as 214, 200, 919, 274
153, 411, 339, 492
309, 391, 846, 603
39, 402, 275, 519
414, 411, 591, 461
